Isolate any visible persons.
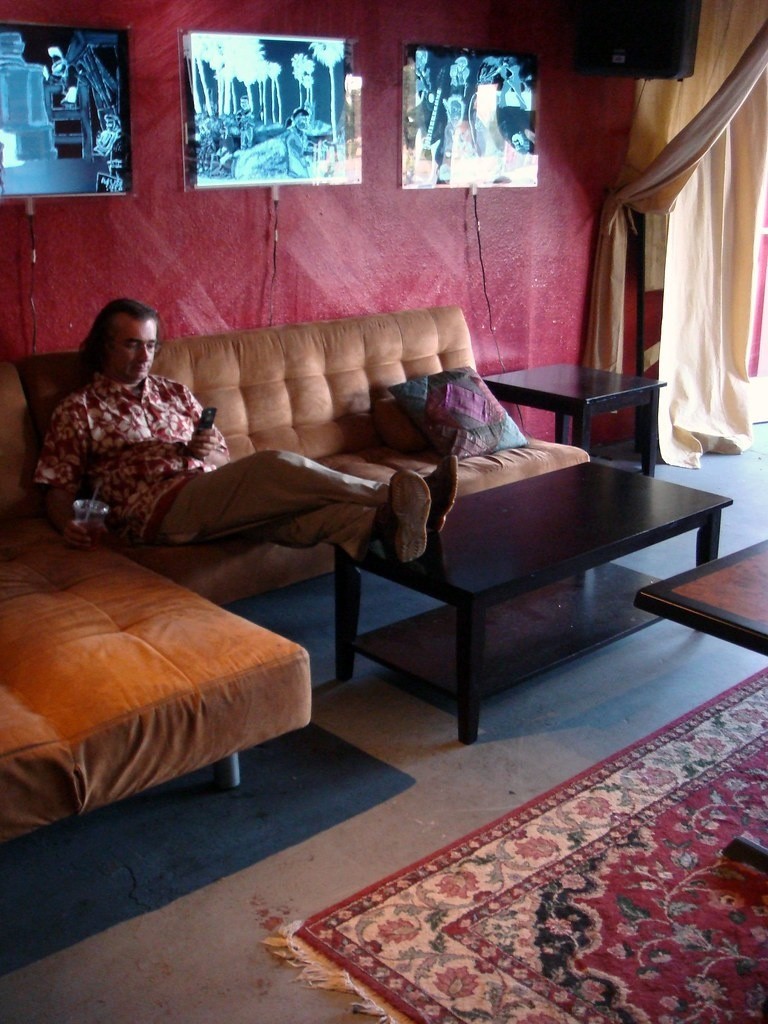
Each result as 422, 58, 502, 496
34, 298, 458, 562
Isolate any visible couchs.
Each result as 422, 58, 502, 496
0, 305, 592, 848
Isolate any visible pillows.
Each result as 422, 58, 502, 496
386, 365, 529, 460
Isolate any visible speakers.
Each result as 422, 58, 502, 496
572, 0, 702, 83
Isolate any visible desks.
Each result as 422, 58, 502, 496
483, 362, 667, 476
635, 541, 768, 872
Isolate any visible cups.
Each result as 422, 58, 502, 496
71, 497, 110, 554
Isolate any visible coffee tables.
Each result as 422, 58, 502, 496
319, 461, 735, 745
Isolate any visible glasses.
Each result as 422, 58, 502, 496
110, 340, 161, 354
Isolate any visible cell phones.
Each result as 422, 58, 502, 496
196, 407, 216, 435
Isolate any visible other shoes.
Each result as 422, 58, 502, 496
423, 455, 459, 534
374, 469, 431, 562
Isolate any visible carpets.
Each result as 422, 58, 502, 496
278, 666, 768, 1024
